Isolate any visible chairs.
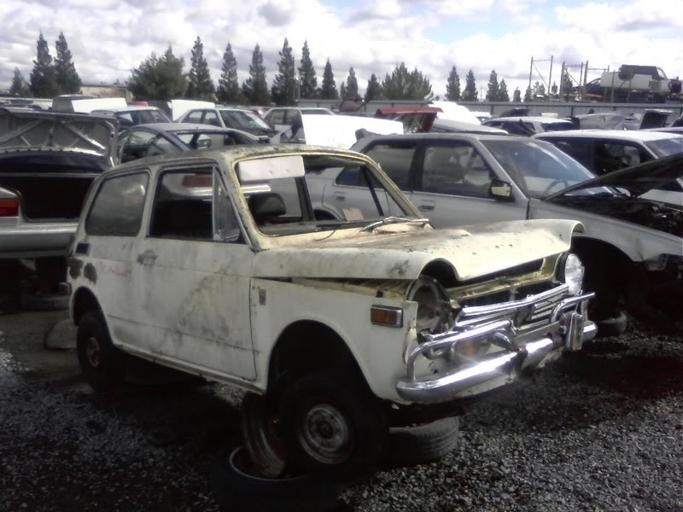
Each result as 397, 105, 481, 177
426, 159, 525, 196
150, 191, 287, 236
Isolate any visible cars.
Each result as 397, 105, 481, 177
0, 93, 683, 259
267, 131, 683, 335
67, 143, 600, 470
